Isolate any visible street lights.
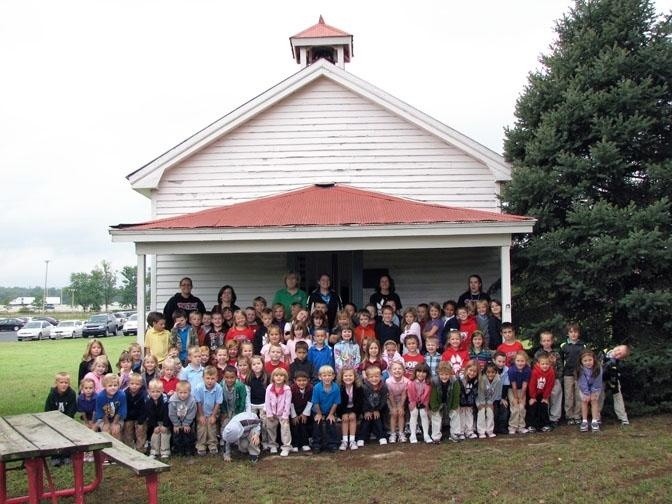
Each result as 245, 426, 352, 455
44, 259, 50, 316
68, 288, 78, 309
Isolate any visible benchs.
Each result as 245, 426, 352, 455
94, 430, 171, 504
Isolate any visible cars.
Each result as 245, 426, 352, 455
36, 317, 59, 325
44, 303, 55, 309
51, 319, 83, 338
17, 321, 54, 341
1, 318, 27, 331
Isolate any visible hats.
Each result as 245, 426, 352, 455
435, 360, 455, 375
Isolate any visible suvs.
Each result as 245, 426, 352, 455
111, 312, 126, 329
123, 313, 149, 335
83, 313, 118, 336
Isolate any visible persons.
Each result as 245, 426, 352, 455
42, 270, 631, 463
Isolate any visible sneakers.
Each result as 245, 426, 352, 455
247, 453, 259, 465
508, 420, 558, 435
260, 439, 364, 456
379, 431, 496, 445
567, 412, 629, 432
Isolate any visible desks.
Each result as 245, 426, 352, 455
0, 410, 112, 504
